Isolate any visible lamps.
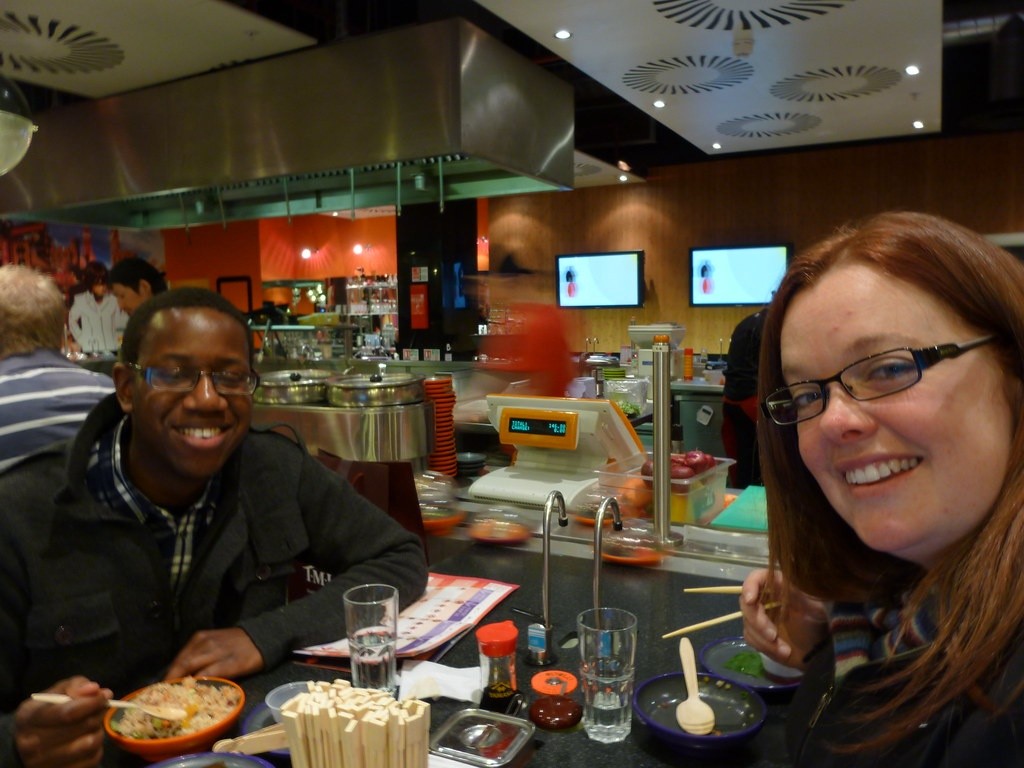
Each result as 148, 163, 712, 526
0, 109, 39, 177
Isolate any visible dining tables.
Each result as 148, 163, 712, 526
89, 534, 810, 768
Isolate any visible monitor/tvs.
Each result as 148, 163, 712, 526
688, 242, 794, 307
486, 393, 651, 490
555, 250, 645, 308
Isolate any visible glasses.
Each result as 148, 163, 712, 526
125, 357, 261, 399
761, 330, 1008, 427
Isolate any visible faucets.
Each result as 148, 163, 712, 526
541, 490, 570, 632
592, 496, 624, 663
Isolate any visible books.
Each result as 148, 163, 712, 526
292, 571, 520, 680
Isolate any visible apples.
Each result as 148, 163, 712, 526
640, 448, 715, 493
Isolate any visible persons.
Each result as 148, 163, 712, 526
0, 256, 170, 471
721, 312, 770, 487
738, 211, 1024, 768
0, 288, 427, 768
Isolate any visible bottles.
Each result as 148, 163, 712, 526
530, 671, 577, 729
476, 621, 520, 715
683, 348, 693, 381
347, 272, 398, 312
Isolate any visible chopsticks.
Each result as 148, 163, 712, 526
662, 586, 781, 640
280, 680, 432, 768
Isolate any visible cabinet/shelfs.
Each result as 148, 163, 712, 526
256, 273, 399, 361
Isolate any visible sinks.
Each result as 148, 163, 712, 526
558, 632, 580, 649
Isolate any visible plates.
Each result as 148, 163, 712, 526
243, 703, 289, 755
424, 377, 487, 480
601, 368, 626, 380
149, 753, 275, 768
593, 550, 664, 564
422, 512, 464, 537
467, 523, 531, 542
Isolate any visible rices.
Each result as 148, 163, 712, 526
111, 675, 243, 740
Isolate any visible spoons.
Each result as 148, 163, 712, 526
675, 638, 716, 734
31, 692, 187, 721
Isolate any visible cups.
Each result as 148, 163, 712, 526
343, 583, 398, 690
577, 607, 637, 743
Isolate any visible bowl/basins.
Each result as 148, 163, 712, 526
266, 681, 312, 725
633, 671, 767, 749
103, 676, 245, 763
699, 635, 803, 696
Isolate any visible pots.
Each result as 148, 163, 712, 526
325, 371, 425, 406
252, 368, 331, 405
